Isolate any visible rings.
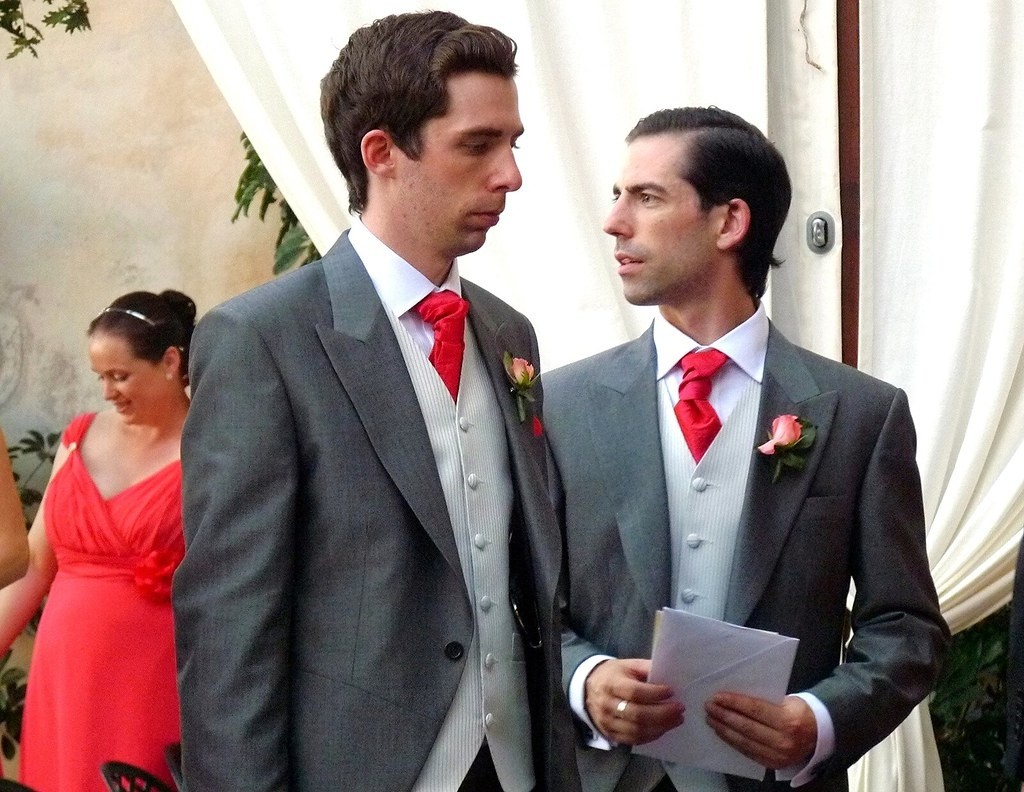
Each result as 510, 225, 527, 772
617, 700, 628, 718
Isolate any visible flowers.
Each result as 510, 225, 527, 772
752, 413, 817, 484
501, 349, 543, 423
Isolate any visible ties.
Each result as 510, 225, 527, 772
672, 351, 728, 463
410, 288, 470, 402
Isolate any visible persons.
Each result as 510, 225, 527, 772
0, 426, 30, 779
541, 107, 951, 792
0, 290, 197, 792
173, 13, 582, 792
